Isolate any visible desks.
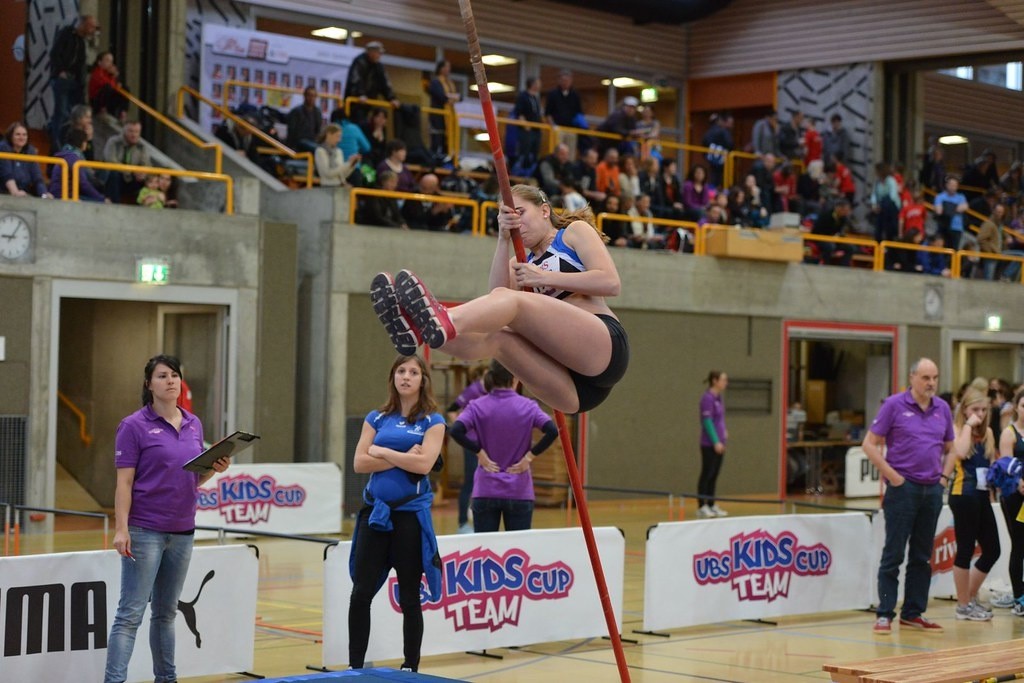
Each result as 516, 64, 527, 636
786, 439, 863, 495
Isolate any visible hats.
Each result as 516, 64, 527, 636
366, 42, 386, 53
623, 95, 642, 107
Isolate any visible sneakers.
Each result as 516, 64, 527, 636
696, 503, 716, 518
873, 617, 891, 634
970, 598, 992, 612
990, 593, 1014, 607
1008, 601, 1024, 616
955, 604, 993, 621
394, 268, 458, 351
900, 616, 944, 631
369, 272, 424, 356
711, 505, 727, 516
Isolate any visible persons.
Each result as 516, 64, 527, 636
103, 354, 230, 683
986, 389, 1024, 617
861, 356, 957, 634
346, 353, 449, 672
446, 365, 558, 533
219, 41, 1024, 284
938, 375, 1024, 502
953, 388, 1001, 620
696, 369, 731, 517
371, 184, 629, 416
0, 15, 177, 209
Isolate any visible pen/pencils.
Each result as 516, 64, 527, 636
125, 551, 136, 561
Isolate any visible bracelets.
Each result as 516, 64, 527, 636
941, 475, 949, 481
965, 423, 973, 429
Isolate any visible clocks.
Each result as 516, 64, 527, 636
923, 284, 945, 321
0, 213, 32, 259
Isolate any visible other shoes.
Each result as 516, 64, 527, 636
457, 525, 475, 533
